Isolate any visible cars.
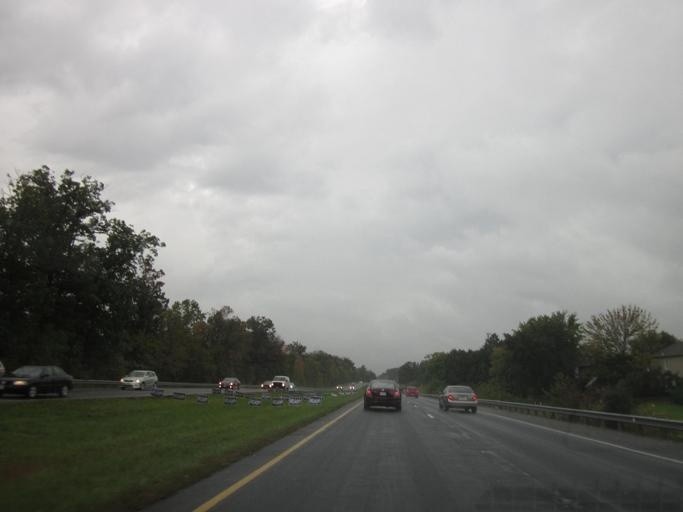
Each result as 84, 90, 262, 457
407, 386, 419, 398
439, 385, 478, 414
1, 363, 74, 398
261, 374, 295, 391
218, 377, 240, 390
364, 377, 401, 412
119, 370, 158, 390
